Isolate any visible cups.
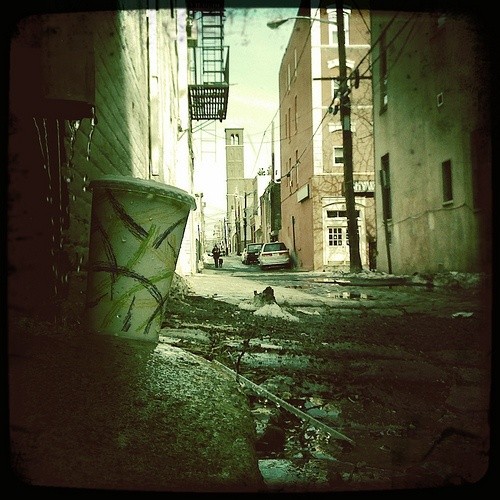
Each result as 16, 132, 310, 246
87, 174, 197, 344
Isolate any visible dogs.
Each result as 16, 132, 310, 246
219, 259, 223, 268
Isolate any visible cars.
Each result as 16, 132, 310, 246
258, 241, 292, 270
241, 248, 247, 264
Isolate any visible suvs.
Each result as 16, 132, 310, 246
245, 243, 263, 264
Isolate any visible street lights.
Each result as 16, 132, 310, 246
267, 16, 365, 275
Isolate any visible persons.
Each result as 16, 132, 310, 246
219, 255, 223, 268
212, 244, 220, 268
